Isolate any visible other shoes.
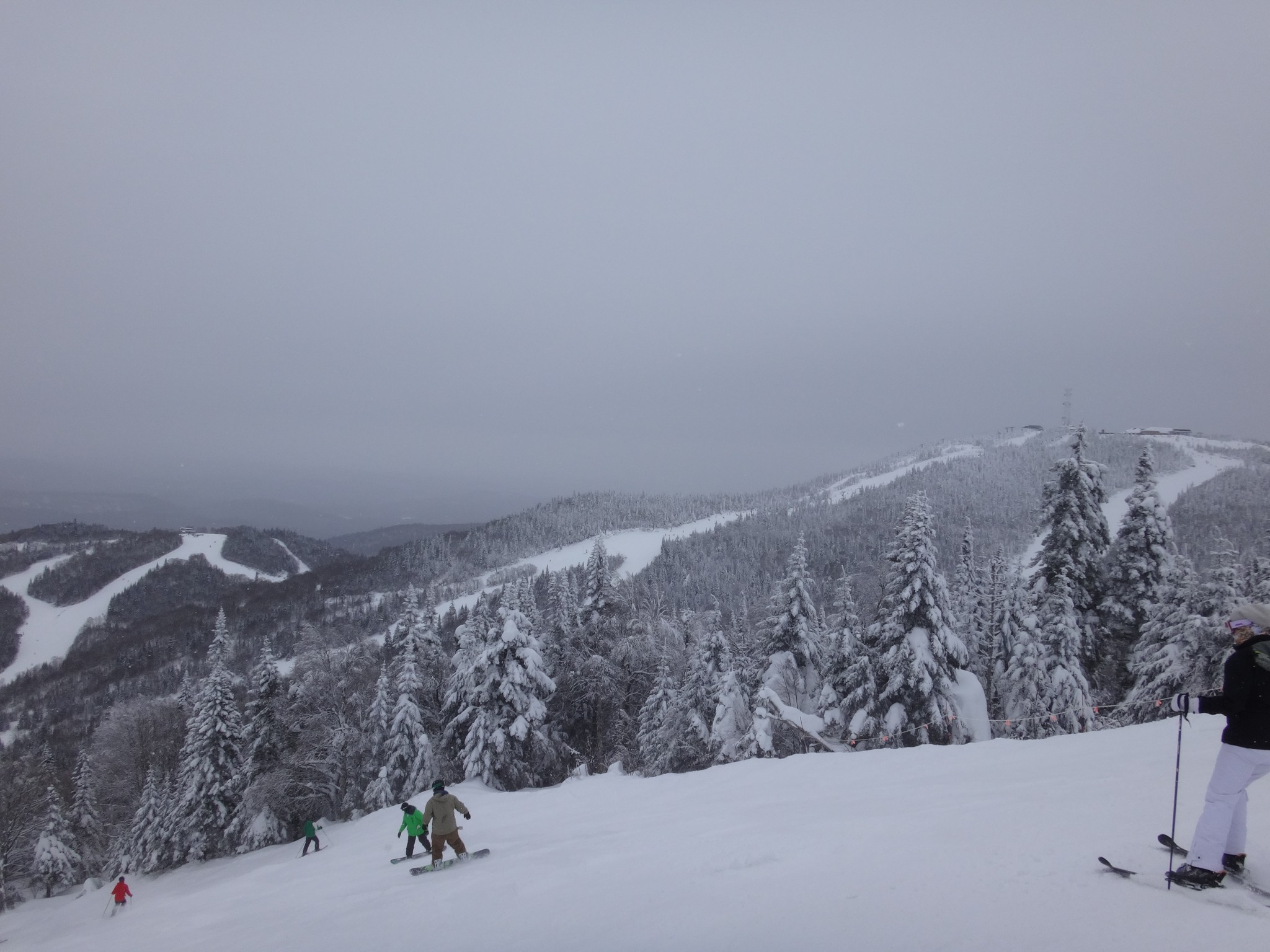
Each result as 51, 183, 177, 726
315, 849, 320, 852
1175, 864, 1223, 886
302, 852, 308, 856
427, 849, 432, 854
407, 853, 412, 858
1221, 853, 1247, 873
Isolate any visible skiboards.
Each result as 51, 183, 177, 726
1098, 833, 1270, 916
296, 845, 328, 859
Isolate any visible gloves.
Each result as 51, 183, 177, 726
422, 824, 428, 830
398, 832, 401, 838
319, 826, 322, 829
464, 812, 470, 820
424, 831, 429, 836
1171, 692, 1199, 714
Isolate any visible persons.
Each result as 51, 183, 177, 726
1169, 602, 1270, 886
398, 802, 433, 859
422, 780, 471, 870
302, 816, 322, 857
111, 876, 133, 918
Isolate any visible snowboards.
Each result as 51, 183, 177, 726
390, 846, 447, 864
409, 848, 490, 876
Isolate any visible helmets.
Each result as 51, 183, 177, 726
401, 802, 409, 811
307, 817, 313, 820
432, 780, 445, 792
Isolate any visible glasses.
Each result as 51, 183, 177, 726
1227, 619, 1236, 635
